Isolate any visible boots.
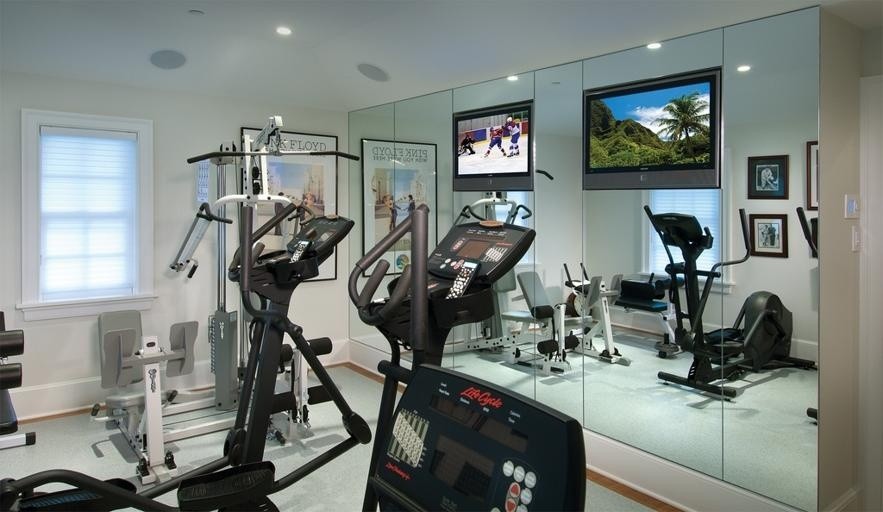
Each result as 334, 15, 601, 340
503, 150, 519, 156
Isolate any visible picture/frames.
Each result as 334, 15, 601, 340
239, 127, 338, 283
362, 139, 438, 278
750, 214, 788, 259
806, 141, 819, 211
747, 155, 789, 199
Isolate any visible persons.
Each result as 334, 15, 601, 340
761, 168, 773, 190
388, 193, 415, 237
457, 131, 475, 157
483, 121, 506, 157
502, 117, 520, 157
761, 224, 776, 246
274, 192, 283, 235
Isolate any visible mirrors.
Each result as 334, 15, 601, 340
347, 7, 821, 511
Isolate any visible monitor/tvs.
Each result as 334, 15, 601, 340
452, 99, 535, 192
583, 70, 721, 189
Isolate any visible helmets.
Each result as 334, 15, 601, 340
507, 117, 513, 122
